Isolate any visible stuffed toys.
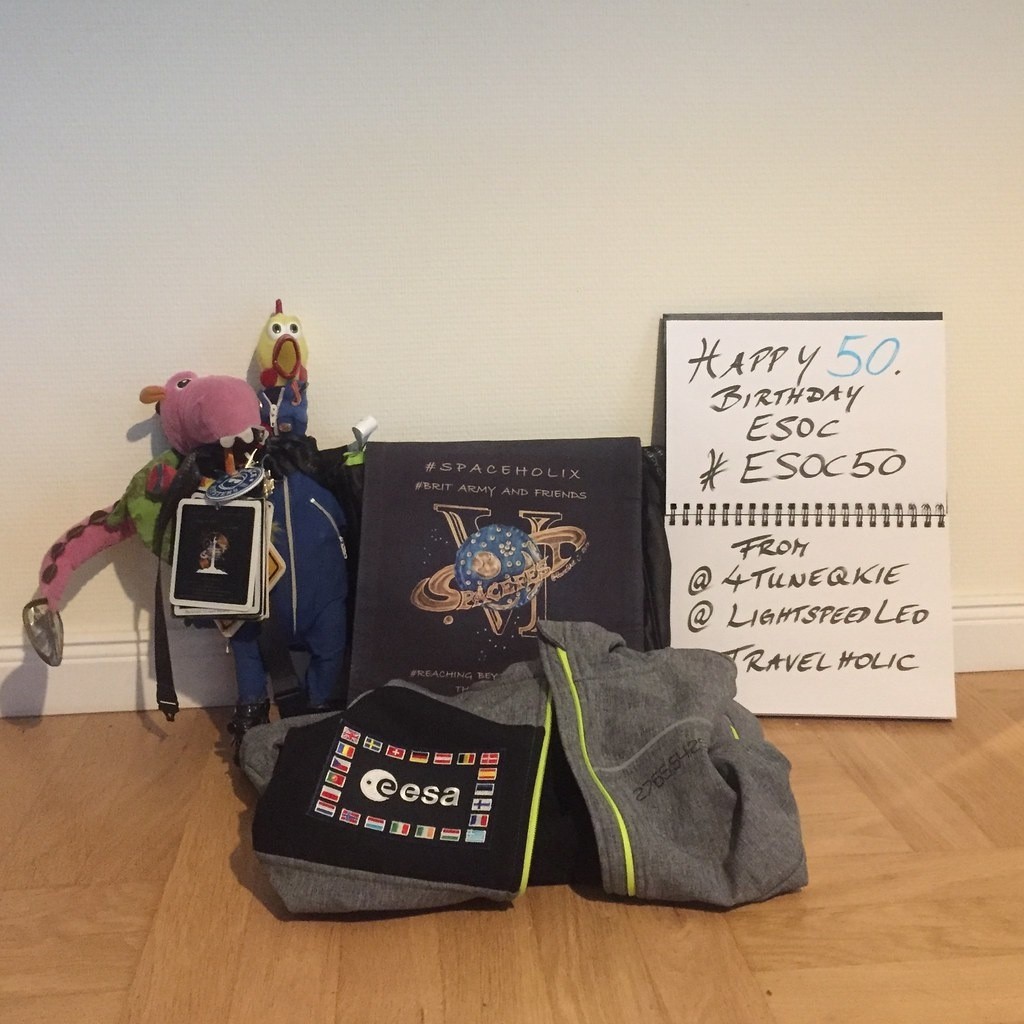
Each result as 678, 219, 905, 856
40, 371, 263, 616
